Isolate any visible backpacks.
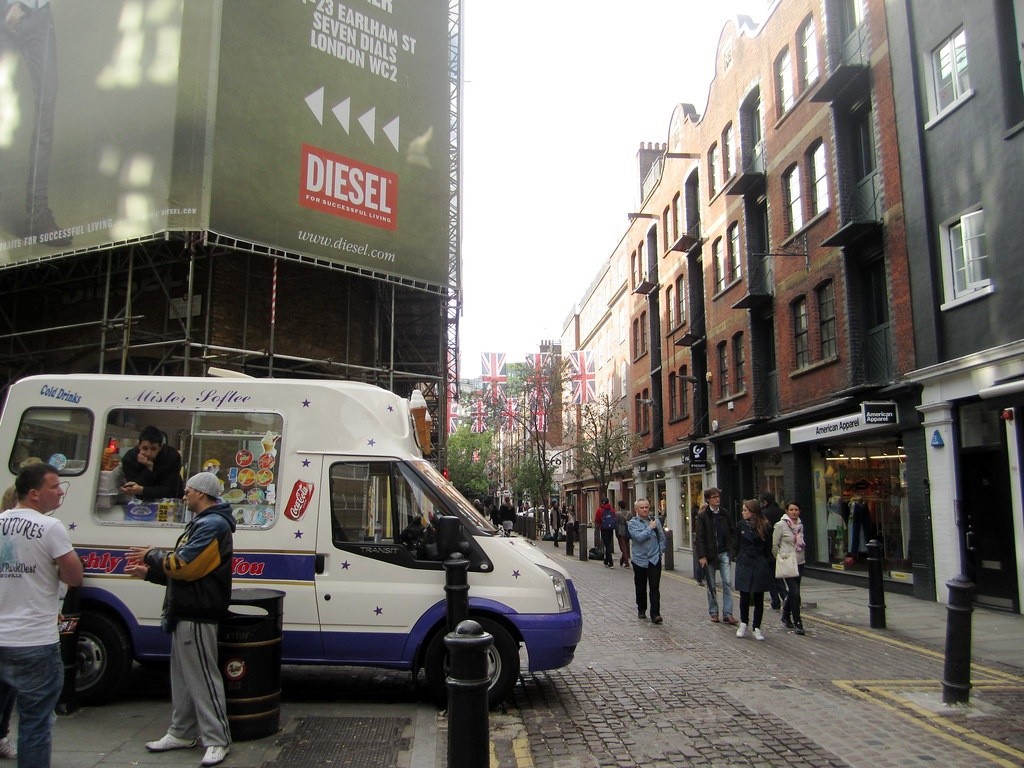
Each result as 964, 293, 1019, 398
617, 510, 631, 539
601, 509, 615, 529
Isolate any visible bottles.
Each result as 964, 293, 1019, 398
157, 498, 183, 523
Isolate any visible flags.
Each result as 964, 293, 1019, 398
447, 349, 597, 460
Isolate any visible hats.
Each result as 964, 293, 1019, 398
187, 472, 220, 497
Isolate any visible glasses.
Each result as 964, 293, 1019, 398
710, 495, 721, 500
184, 489, 196, 496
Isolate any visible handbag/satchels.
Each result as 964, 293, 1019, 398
775, 553, 799, 578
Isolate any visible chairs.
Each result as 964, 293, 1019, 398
331, 513, 346, 541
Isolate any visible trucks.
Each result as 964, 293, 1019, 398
1, 367, 583, 703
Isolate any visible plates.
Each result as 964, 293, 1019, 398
128, 504, 157, 521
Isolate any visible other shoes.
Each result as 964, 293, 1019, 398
638, 612, 646, 618
710, 614, 719, 622
651, 616, 663, 623
696, 581, 705, 587
781, 616, 804, 635
604, 559, 614, 568
620, 559, 630, 568
724, 615, 738, 623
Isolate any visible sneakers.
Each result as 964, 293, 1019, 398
145, 734, 197, 750
736, 622, 747, 638
751, 627, 764, 641
201, 745, 229, 766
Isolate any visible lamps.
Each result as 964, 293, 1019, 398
680, 451, 689, 462
638, 462, 646, 472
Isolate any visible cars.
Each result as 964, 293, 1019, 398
516, 506, 552, 521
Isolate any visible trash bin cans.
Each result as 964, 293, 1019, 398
216, 588, 286, 741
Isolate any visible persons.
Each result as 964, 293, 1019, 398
696, 504, 707, 586
124, 472, 236, 765
595, 498, 616, 568
757, 492, 789, 610
736, 499, 774, 640
615, 500, 633, 568
771, 502, 806, 635
119, 425, 184, 502
628, 499, 667, 623
0, 463, 84, 768
472, 495, 577, 547
695, 487, 740, 625
825, 487, 847, 532
